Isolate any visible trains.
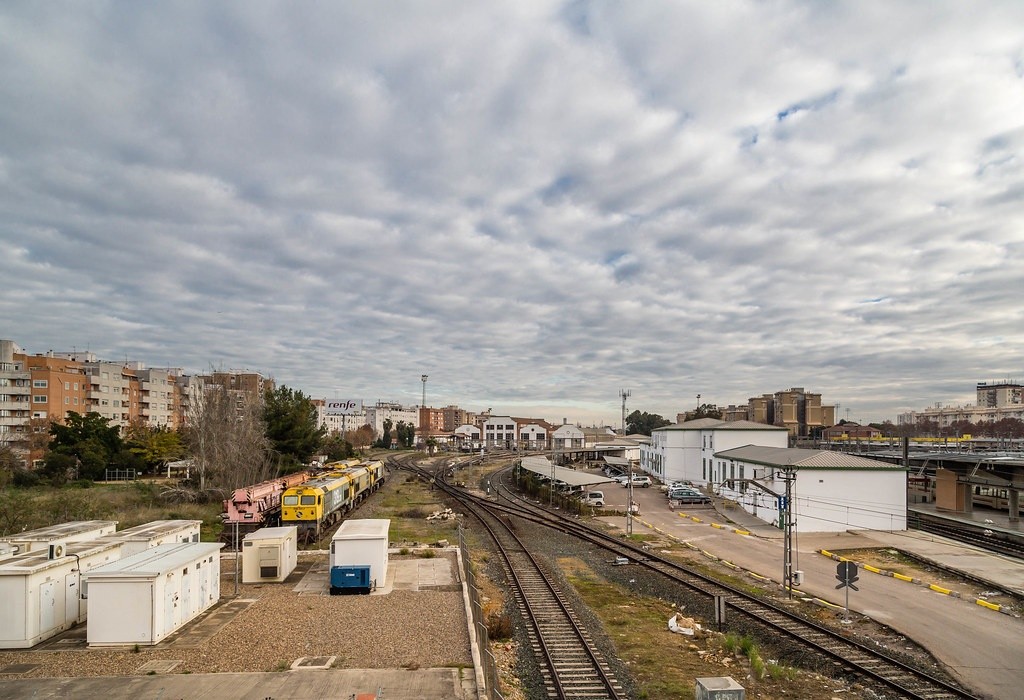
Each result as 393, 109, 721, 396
275, 458, 385, 545
219, 457, 362, 545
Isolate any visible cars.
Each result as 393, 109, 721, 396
610, 472, 638, 483
533, 472, 585, 499
659, 480, 712, 505
567, 490, 606, 508
600, 463, 625, 478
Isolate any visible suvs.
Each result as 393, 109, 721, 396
620, 475, 652, 488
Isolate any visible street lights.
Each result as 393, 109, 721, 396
488, 407, 492, 464
